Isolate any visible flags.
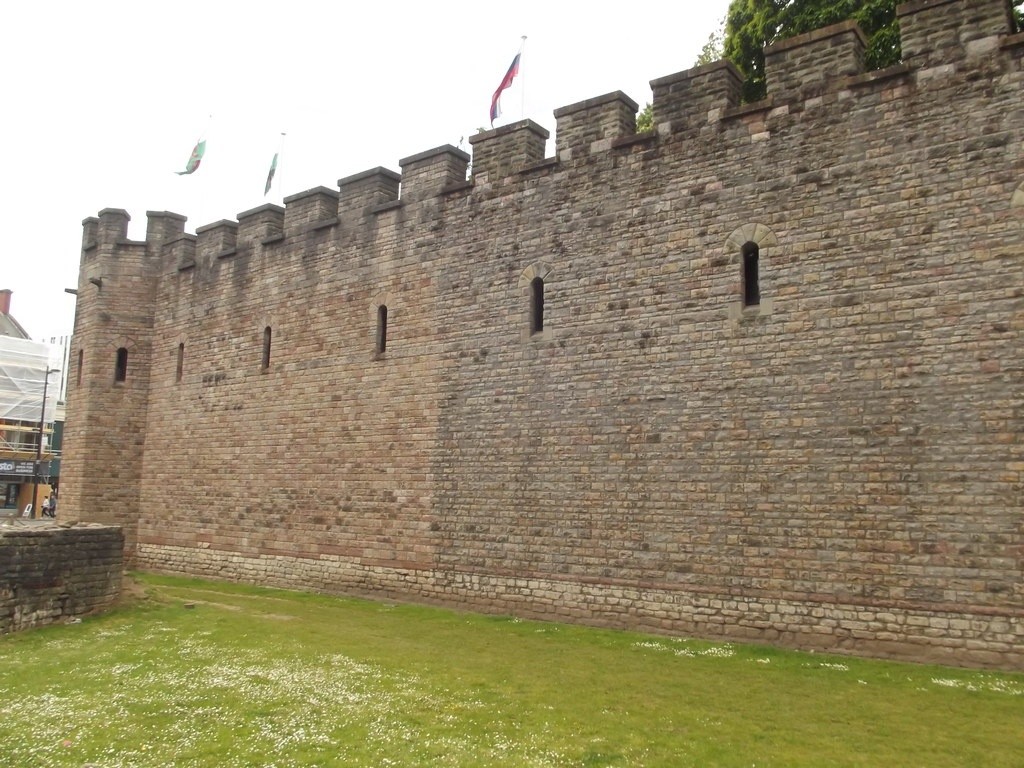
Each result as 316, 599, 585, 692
175, 140, 206, 175
490, 42, 523, 126
264, 152, 278, 195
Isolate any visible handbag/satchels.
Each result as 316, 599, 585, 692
41, 506, 43, 508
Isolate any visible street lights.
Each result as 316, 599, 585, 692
31, 369, 60, 518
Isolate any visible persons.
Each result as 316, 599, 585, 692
41, 496, 49, 517
49, 495, 56, 517
